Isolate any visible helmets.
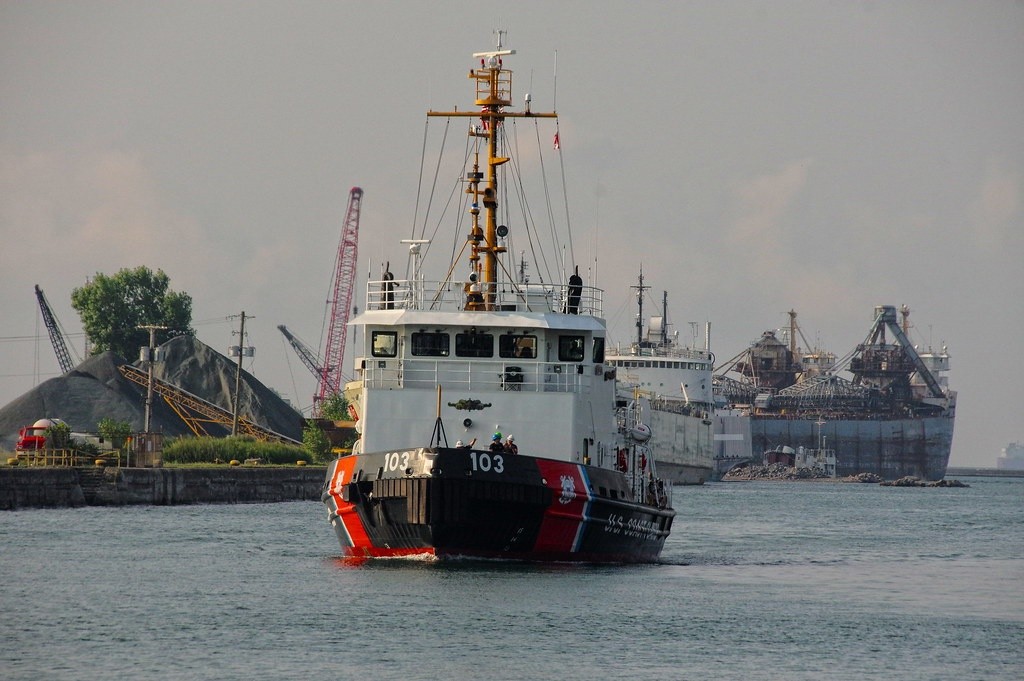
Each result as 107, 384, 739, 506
507, 435, 515, 441
493, 432, 503, 440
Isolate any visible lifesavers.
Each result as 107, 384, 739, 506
620, 448, 629, 472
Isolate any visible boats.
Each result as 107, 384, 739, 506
321, 28, 677, 564
711, 304, 958, 481
605, 260, 716, 484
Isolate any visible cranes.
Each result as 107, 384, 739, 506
312, 186, 361, 419
118, 364, 303, 447
34, 284, 73, 375
278, 323, 355, 404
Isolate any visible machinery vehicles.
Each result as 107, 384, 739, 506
16, 417, 70, 464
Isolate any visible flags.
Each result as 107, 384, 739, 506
553, 131, 560, 151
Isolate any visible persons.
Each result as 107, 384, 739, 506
569, 341, 582, 355
456, 438, 477, 449
489, 432, 519, 455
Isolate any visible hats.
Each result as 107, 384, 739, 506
455, 441, 464, 447
